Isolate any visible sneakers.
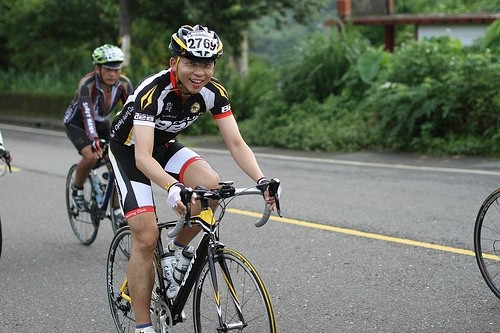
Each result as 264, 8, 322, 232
70, 184, 86, 212
111, 212, 127, 224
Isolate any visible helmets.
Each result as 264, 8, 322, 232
169, 24, 223, 58
91, 43, 124, 64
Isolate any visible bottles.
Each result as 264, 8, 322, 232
161, 248, 180, 299
172, 246, 194, 286
99, 171, 109, 197
93, 173, 103, 203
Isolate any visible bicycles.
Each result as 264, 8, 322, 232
66, 139, 159, 258
105, 181, 277, 333
0, 148, 11, 258
473, 188, 500, 299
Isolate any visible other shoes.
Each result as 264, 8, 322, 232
135, 325, 157, 333
166, 242, 185, 268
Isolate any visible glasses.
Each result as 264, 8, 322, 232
178, 59, 214, 70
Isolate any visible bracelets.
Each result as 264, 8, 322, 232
165, 180, 179, 191
257, 176, 266, 184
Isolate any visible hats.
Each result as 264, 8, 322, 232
102, 63, 122, 70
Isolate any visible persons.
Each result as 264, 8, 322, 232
102, 25, 282, 333
63, 44, 133, 228
